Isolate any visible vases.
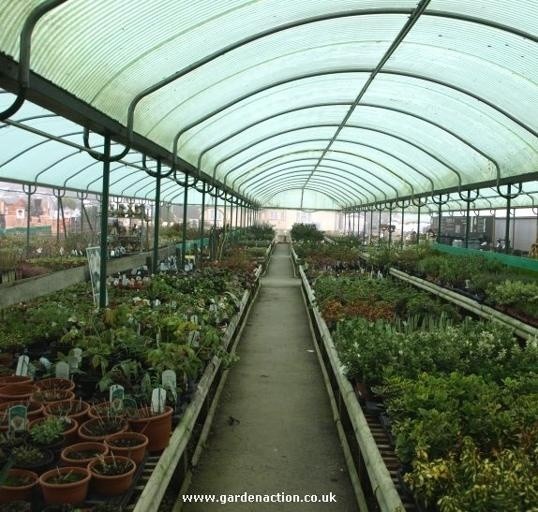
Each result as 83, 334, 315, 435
0, 372, 174, 505
